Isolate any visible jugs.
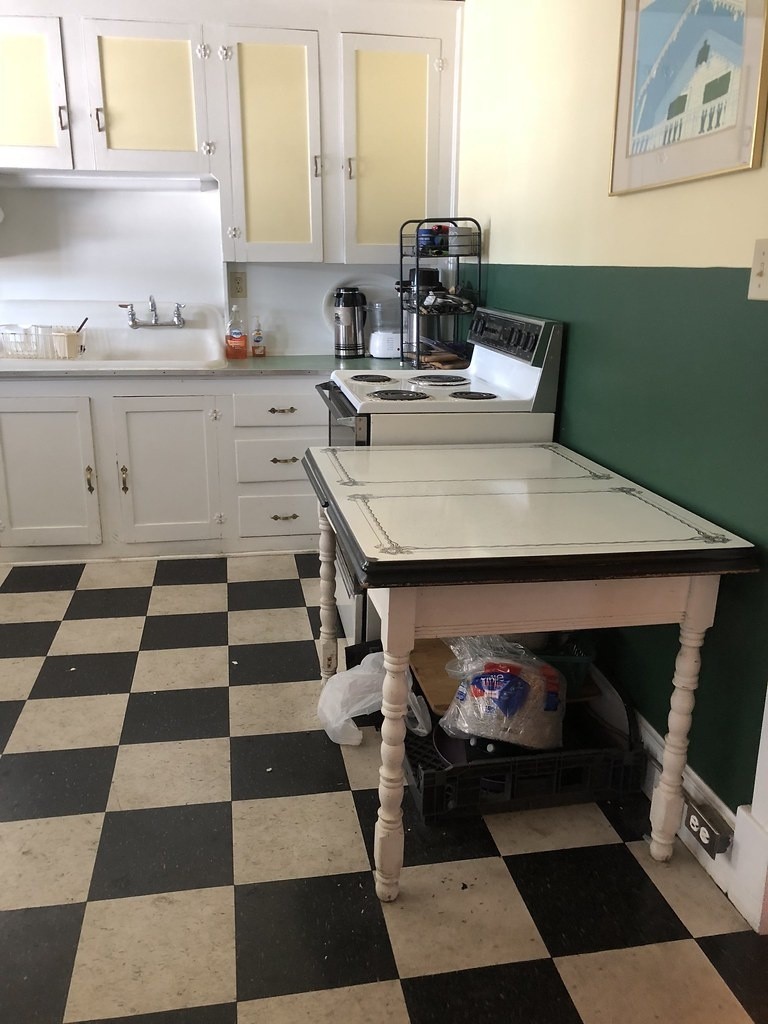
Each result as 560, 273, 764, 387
334, 287, 367, 358
396, 268, 443, 354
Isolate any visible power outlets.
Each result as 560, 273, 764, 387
230, 272, 247, 298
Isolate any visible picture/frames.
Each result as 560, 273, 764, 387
606, 0, 768, 197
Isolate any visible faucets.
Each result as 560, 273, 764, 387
148, 294, 159, 325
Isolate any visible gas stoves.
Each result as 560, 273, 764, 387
332, 307, 563, 415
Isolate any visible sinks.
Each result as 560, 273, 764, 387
85, 327, 221, 366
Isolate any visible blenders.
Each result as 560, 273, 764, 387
369, 300, 406, 359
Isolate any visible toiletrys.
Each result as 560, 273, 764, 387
250, 314, 266, 357
224, 305, 248, 360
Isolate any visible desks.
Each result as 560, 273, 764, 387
299, 439, 768, 905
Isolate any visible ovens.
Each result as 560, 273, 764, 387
315, 382, 555, 645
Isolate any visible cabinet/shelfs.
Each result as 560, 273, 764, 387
216, 393, 330, 556
0, 377, 225, 565
398, 216, 482, 370
0, 1, 213, 180
207, 0, 464, 270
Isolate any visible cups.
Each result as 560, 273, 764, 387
418, 229, 434, 246
31, 325, 52, 352
449, 227, 472, 254
53, 332, 80, 358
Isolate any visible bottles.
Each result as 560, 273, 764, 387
226, 306, 247, 358
251, 316, 265, 356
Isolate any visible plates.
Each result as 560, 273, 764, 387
322, 271, 401, 334
